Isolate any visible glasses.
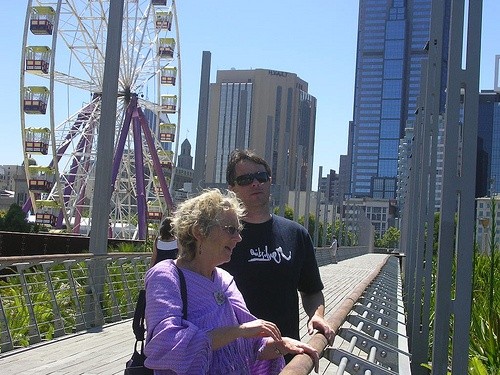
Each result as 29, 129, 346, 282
222, 225, 241, 236
231, 172, 269, 186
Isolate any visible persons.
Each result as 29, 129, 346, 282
215, 148, 336, 366
142, 187, 318, 375
330, 234, 338, 264
149, 217, 179, 268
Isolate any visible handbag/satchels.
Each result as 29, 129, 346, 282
124, 264, 188, 375
